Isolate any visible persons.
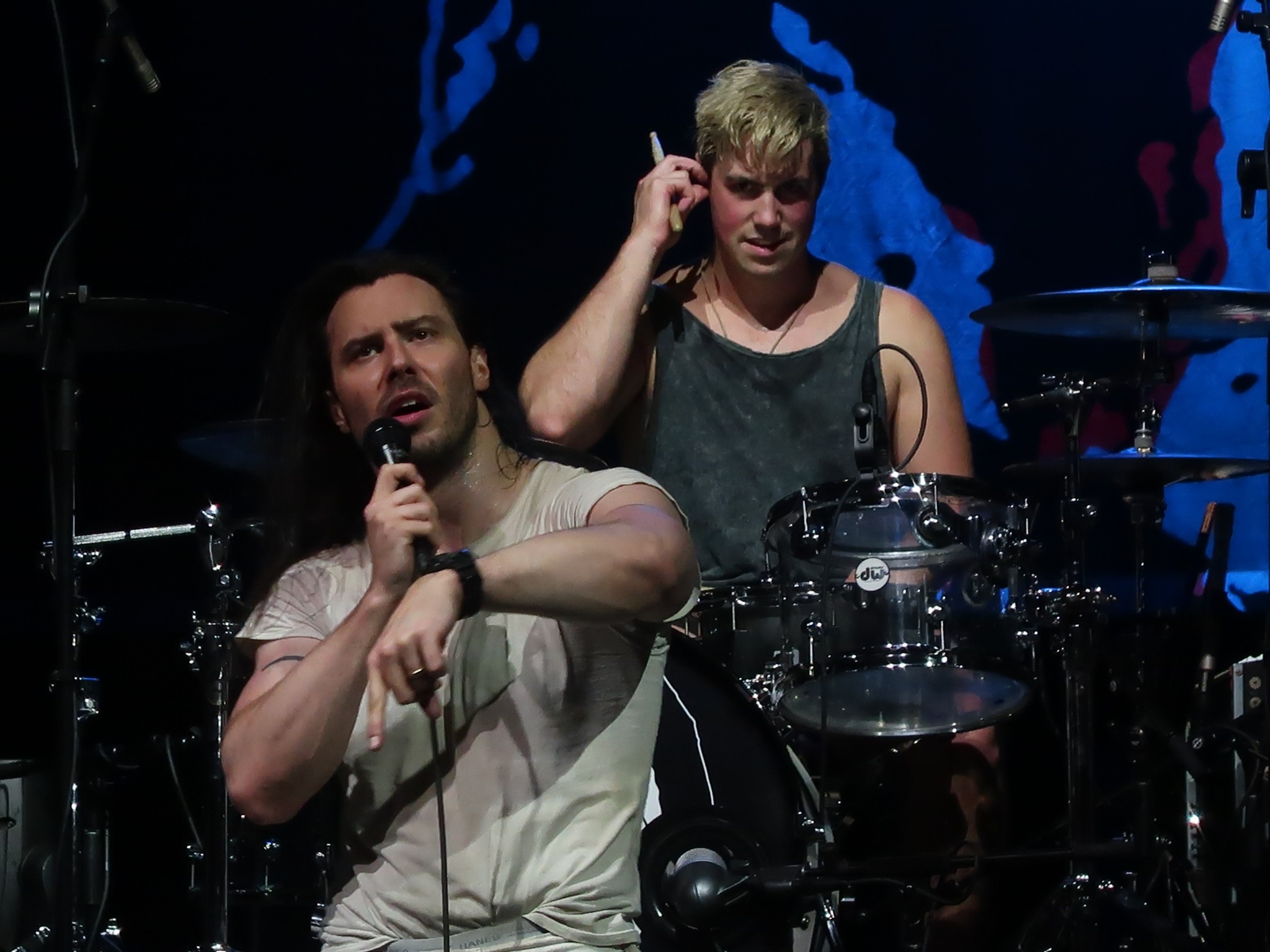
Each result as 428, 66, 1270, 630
221, 258, 704, 952
513, 54, 1004, 952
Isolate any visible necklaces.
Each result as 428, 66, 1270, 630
703, 251, 814, 352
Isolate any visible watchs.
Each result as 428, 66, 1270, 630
425, 545, 483, 619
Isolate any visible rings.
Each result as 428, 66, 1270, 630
406, 667, 425, 682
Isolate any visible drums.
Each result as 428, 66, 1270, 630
640, 615, 843, 952
761, 467, 1040, 741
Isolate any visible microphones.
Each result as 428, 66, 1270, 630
862, 358, 887, 467
363, 417, 432, 570
102, 1, 161, 94
1000, 378, 1114, 418
666, 847, 733, 935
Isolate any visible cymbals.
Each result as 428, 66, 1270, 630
992, 447, 1269, 483
967, 277, 1270, 340
